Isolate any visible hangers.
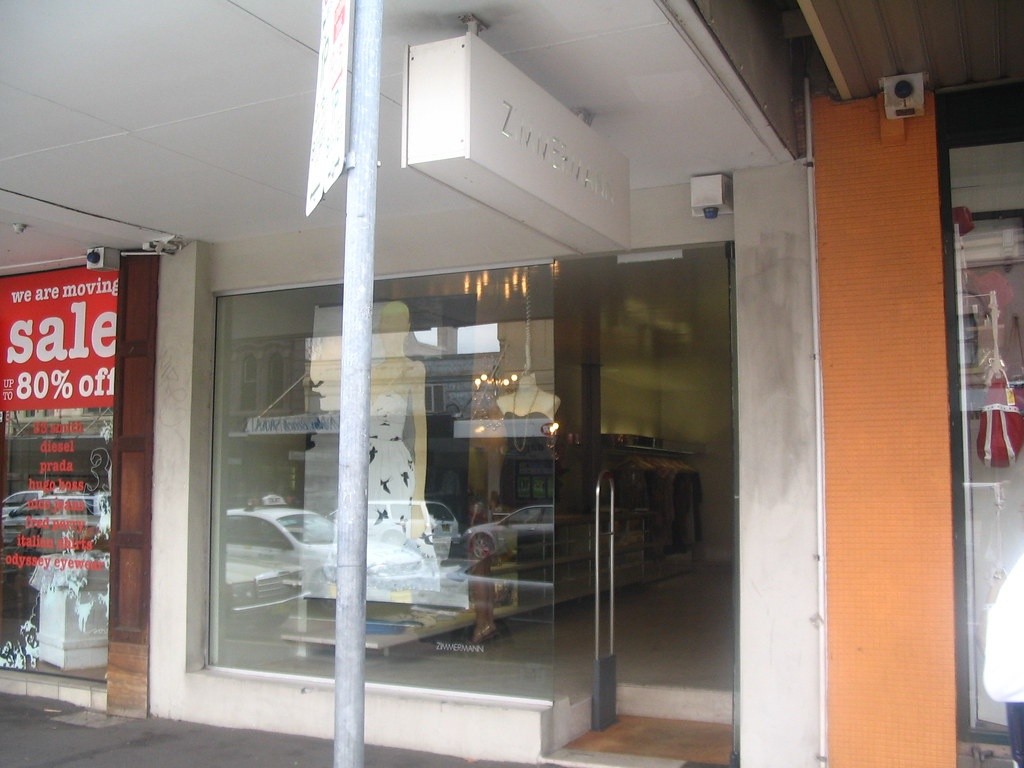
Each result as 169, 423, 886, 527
616, 454, 699, 474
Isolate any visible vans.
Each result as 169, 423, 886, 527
323, 501, 462, 560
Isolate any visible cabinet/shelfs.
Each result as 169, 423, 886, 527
307, 434, 704, 649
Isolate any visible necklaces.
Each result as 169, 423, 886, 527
512, 386, 540, 452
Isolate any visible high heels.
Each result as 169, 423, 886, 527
465, 625, 499, 647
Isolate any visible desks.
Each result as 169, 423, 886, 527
29, 550, 109, 671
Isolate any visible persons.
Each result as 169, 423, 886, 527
321, 301, 441, 593
497, 372, 561, 509
463, 532, 500, 645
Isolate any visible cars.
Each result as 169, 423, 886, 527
1, 489, 111, 556
224, 495, 434, 613
221, 555, 312, 639
462, 502, 555, 560
